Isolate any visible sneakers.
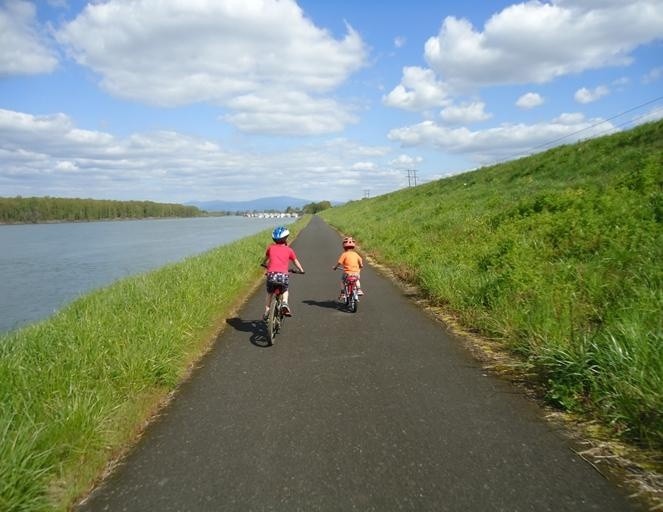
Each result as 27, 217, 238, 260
339, 293, 347, 301
281, 301, 292, 319
357, 290, 364, 297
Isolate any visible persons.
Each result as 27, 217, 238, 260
260, 226, 305, 321
332, 237, 364, 301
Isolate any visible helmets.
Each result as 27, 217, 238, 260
272, 226, 290, 239
342, 236, 356, 248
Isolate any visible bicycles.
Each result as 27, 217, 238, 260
329, 264, 359, 315
257, 263, 305, 347
